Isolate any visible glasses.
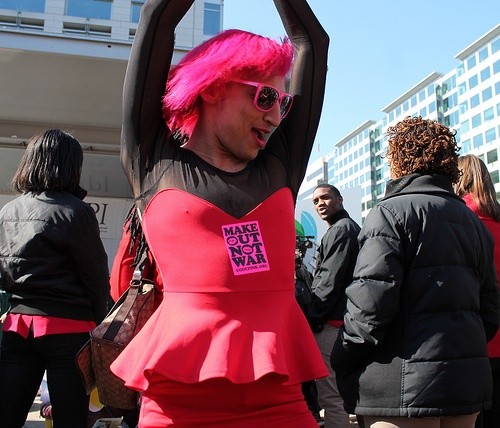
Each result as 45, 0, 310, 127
230, 78, 293, 118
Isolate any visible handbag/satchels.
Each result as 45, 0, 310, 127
75, 277, 156, 409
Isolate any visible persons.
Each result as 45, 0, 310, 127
0, 116, 500, 428
120, 0, 331, 428
